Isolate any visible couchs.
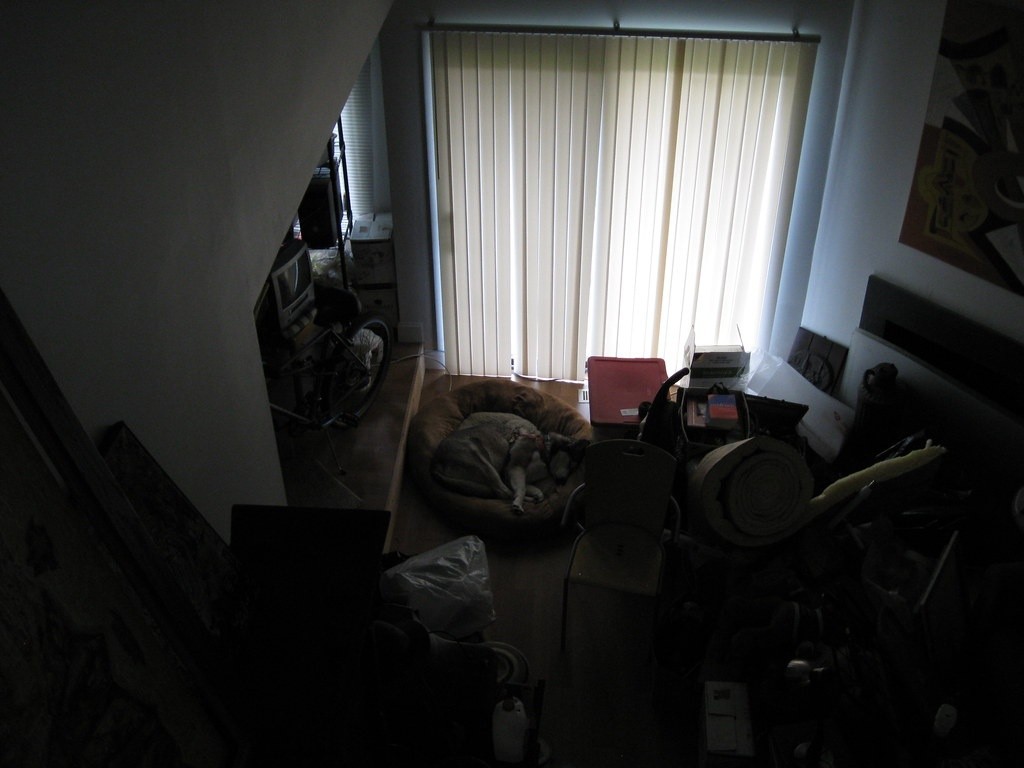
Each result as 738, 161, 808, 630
962, 560, 1024, 768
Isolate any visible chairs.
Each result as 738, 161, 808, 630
560, 439, 681, 668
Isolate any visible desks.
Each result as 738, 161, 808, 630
263, 325, 330, 411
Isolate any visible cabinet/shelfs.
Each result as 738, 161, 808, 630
768, 720, 854, 768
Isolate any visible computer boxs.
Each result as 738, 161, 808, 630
298, 161, 343, 250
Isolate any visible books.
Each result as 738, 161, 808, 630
691, 352, 745, 377
687, 391, 738, 433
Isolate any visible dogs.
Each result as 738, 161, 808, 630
429, 411, 592, 515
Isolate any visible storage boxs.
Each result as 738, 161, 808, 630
349, 211, 396, 285
356, 287, 399, 342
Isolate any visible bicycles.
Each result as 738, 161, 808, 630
262, 288, 392, 476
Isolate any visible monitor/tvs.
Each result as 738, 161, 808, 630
253, 239, 317, 339
